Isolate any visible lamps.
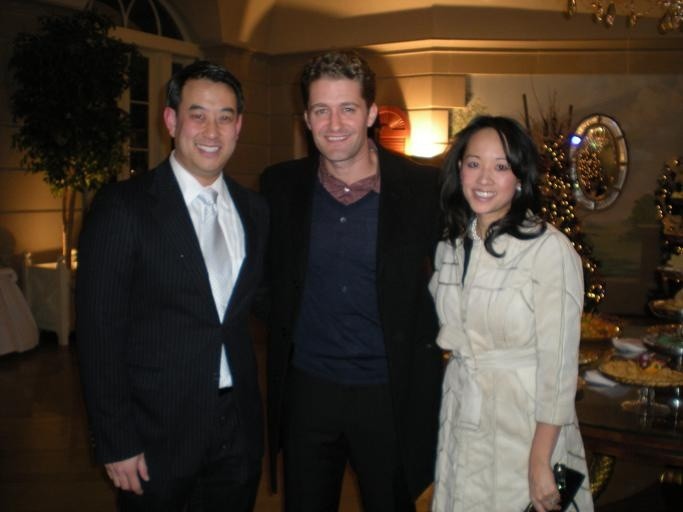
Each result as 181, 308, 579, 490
564, 0, 683, 34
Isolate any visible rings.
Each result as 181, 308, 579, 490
550, 496, 557, 504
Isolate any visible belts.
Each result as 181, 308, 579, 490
216, 386, 235, 399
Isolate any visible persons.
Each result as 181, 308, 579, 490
74, 61, 270, 512
256, 47, 442, 512
424, 115, 596, 512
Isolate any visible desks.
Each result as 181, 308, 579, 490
575, 310, 682, 469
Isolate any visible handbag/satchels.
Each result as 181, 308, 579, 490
522, 460, 587, 512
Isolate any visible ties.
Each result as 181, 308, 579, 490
194, 184, 237, 386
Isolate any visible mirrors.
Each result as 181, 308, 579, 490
571, 114, 627, 211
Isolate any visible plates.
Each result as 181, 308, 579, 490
570, 293, 683, 417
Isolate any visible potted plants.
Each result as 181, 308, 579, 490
6, 10, 142, 346
